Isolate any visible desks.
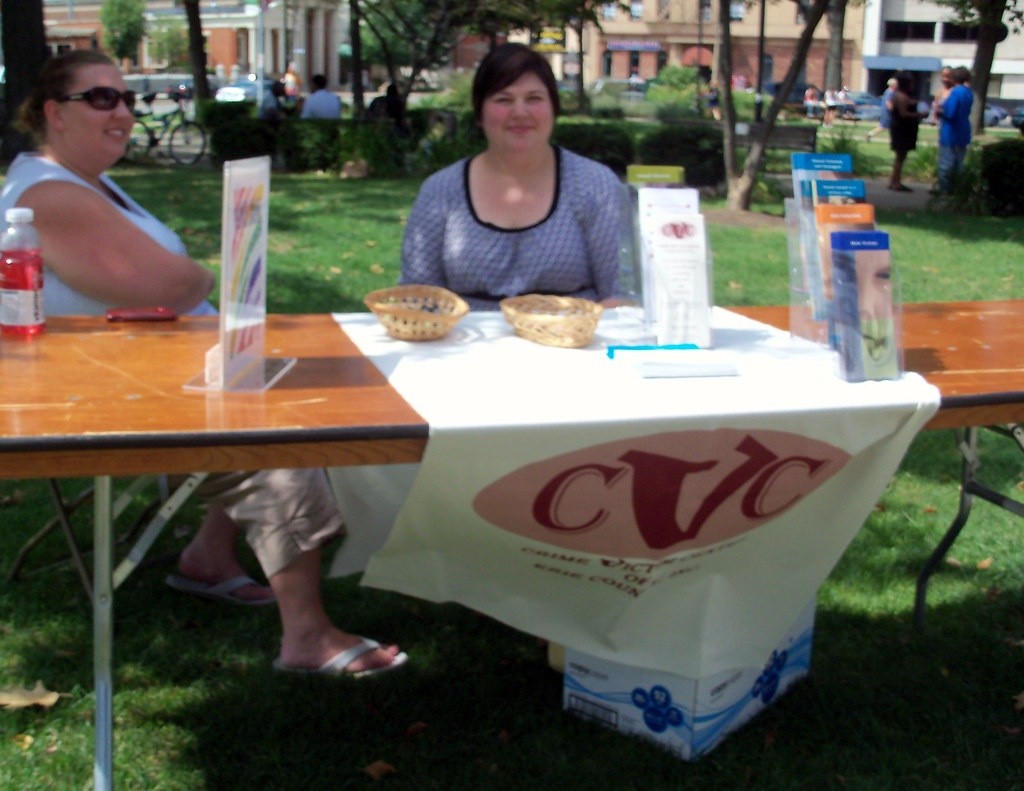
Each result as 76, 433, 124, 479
0, 300, 1024, 791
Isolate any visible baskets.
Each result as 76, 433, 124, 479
498, 292, 604, 349
361, 283, 472, 342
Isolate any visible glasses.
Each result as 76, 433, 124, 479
56, 86, 138, 112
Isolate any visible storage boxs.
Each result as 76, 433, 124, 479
563, 585, 819, 763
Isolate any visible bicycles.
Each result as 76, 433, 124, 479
123, 84, 207, 168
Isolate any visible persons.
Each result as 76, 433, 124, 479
866, 77, 929, 192
0, 49, 407, 681
370, 83, 397, 115
930, 66, 979, 209
804, 86, 849, 128
260, 60, 342, 120
399, 44, 635, 316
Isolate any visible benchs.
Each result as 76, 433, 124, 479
247, 115, 395, 171
659, 114, 817, 174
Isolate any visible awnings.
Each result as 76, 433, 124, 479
683, 47, 716, 69
863, 56, 944, 72
606, 42, 665, 51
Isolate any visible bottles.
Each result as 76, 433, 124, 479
0, 207, 46, 334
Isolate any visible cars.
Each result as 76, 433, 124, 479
140, 70, 300, 122
915, 95, 1024, 133
555, 73, 883, 123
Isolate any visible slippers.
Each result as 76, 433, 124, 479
268, 636, 410, 682
165, 567, 276, 606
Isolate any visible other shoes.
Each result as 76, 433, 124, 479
888, 185, 915, 193
